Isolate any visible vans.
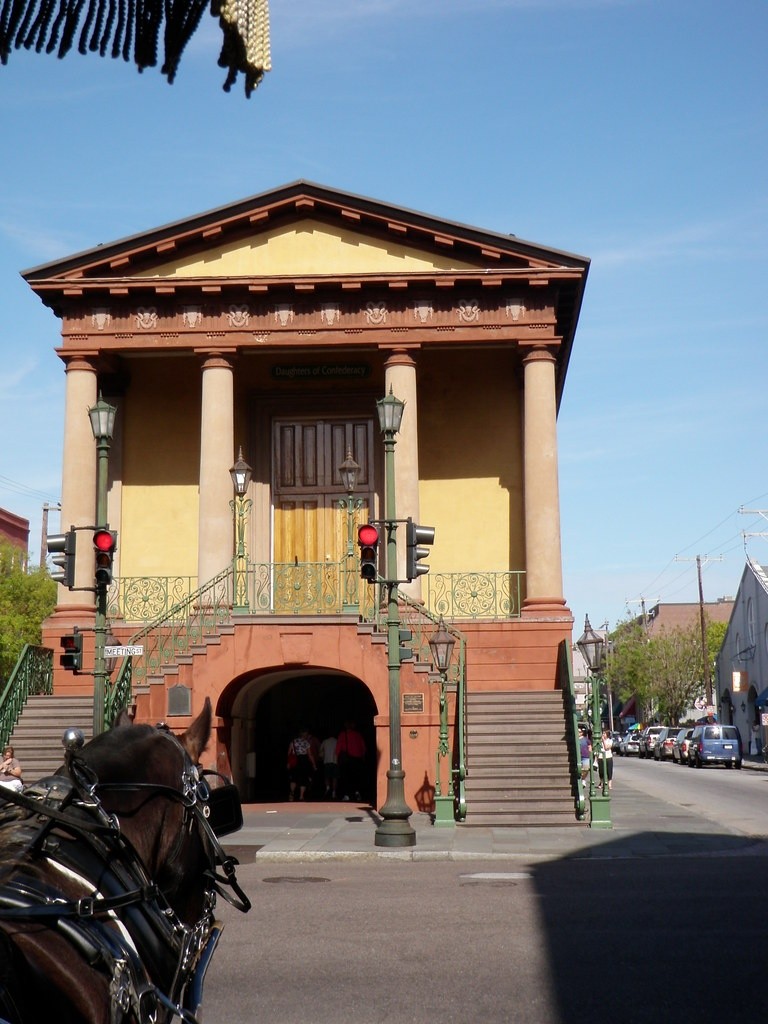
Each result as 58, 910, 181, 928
670, 728, 696, 765
652, 727, 679, 761
687, 724, 743, 770
632, 725, 665, 759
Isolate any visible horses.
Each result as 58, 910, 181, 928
0, 694, 221, 1024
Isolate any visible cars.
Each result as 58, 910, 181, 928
619, 731, 642, 757
608, 731, 625, 755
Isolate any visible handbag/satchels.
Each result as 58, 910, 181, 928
593, 756, 598, 772
287, 739, 298, 769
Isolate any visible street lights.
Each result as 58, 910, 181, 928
338, 442, 363, 562
429, 612, 463, 829
228, 444, 251, 564
87, 387, 119, 739
375, 384, 420, 847
576, 612, 616, 830
102, 620, 123, 735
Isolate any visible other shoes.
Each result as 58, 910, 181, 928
331, 792, 336, 799
301, 797, 304, 800
342, 795, 350, 801
355, 792, 361, 802
325, 785, 330, 795
289, 793, 294, 801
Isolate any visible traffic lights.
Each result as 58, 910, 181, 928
92, 529, 118, 588
357, 522, 379, 580
61, 635, 83, 671
47, 531, 76, 588
406, 517, 435, 582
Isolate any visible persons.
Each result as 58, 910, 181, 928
0, 746, 22, 792
579, 730, 592, 786
595, 730, 613, 789
287, 718, 366, 802
752, 720, 761, 754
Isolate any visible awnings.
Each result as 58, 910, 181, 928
753, 686, 768, 706
613, 697, 636, 718
